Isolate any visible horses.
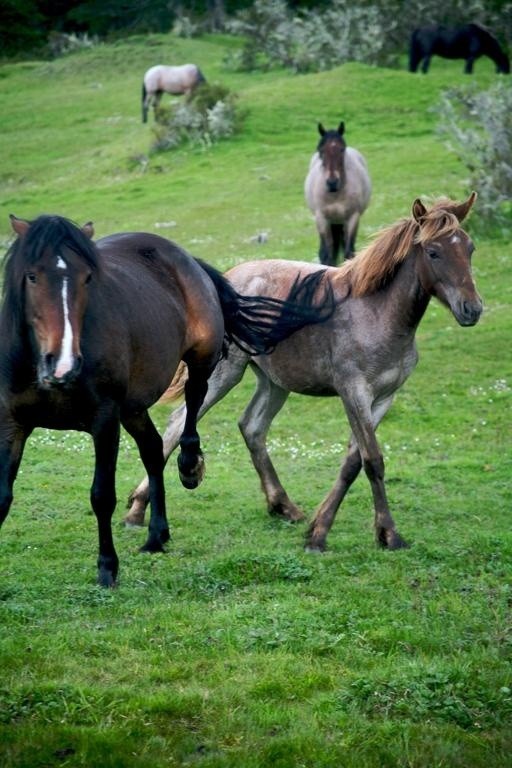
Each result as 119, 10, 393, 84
116, 189, 484, 551
303, 120, 374, 264
139, 62, 208, 123
408, 21, 512, 75
0, 212, 352, 588
